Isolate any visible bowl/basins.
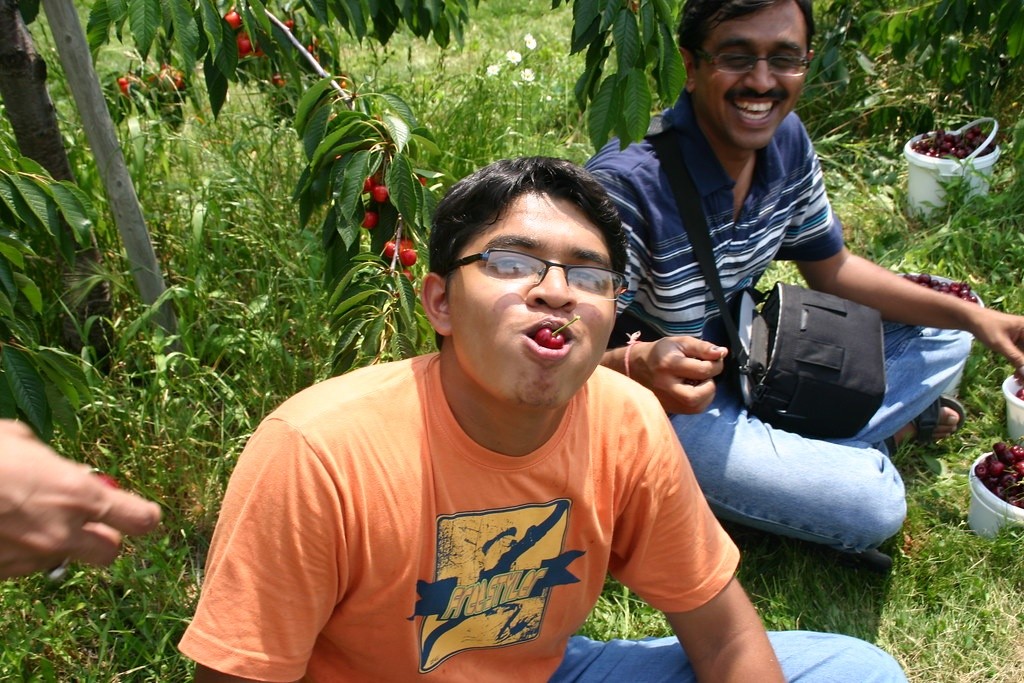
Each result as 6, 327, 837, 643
968, 452, 1024, 537
1002, 375, 1024, 447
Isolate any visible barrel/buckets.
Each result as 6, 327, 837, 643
896, 273, 983, 342
903, 118, 1001, 221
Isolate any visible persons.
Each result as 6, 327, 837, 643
580, 1, 1024, 563
0, 419, 162, 578
177, 155, 907, 683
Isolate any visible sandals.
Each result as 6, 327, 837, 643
883, 395, 966, 459
788, 534, 893, 569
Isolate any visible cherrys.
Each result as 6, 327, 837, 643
534, 315, 580, 350
225, 8, 426, 294
96, 472, 120, 490
1016, 385, 1024, 401
902, 271, 977, 304
975, 436, 1024, 508
912, 124, 997, 159
117, 63, 183, 98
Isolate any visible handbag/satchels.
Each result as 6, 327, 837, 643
722, 279, 886, 440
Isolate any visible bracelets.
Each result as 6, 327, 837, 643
624, 331, 641, 378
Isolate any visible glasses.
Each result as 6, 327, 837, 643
447, 246, 629, 301
696, 43, 810, 76
